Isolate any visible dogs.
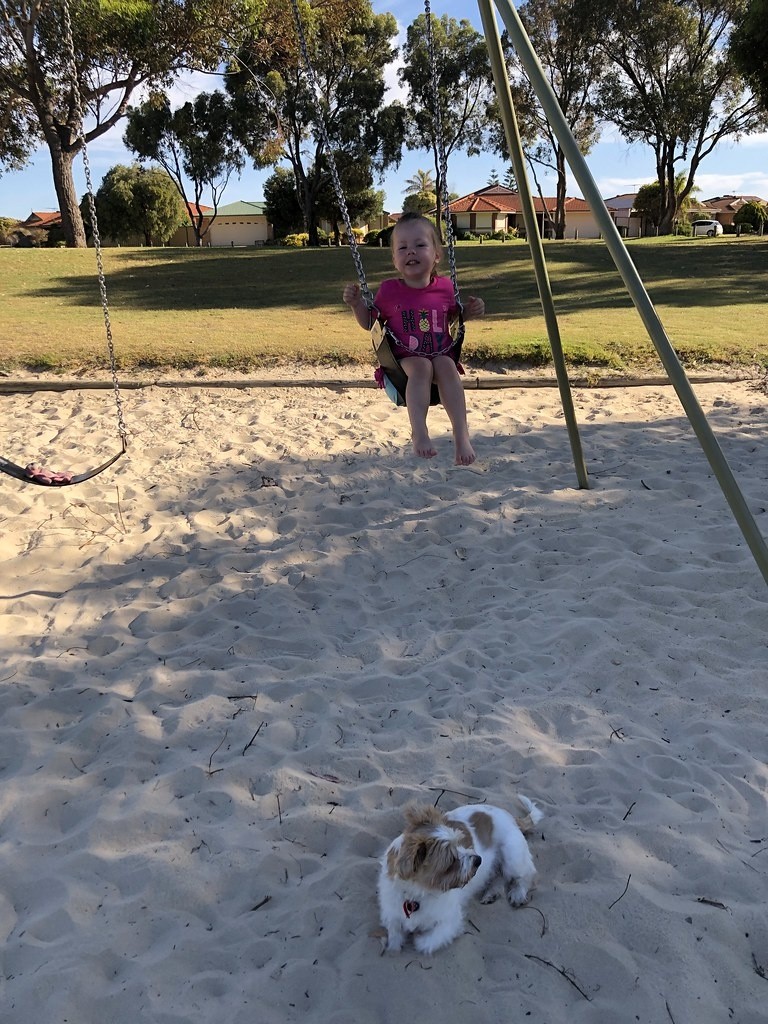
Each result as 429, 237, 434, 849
377, 793, 544, 956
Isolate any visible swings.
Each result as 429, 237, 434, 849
284, 0, 469, 406
1, 0, 129, 488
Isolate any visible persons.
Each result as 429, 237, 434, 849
342, 212, 485, 466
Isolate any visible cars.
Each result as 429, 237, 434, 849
690, 220, 723, 237
